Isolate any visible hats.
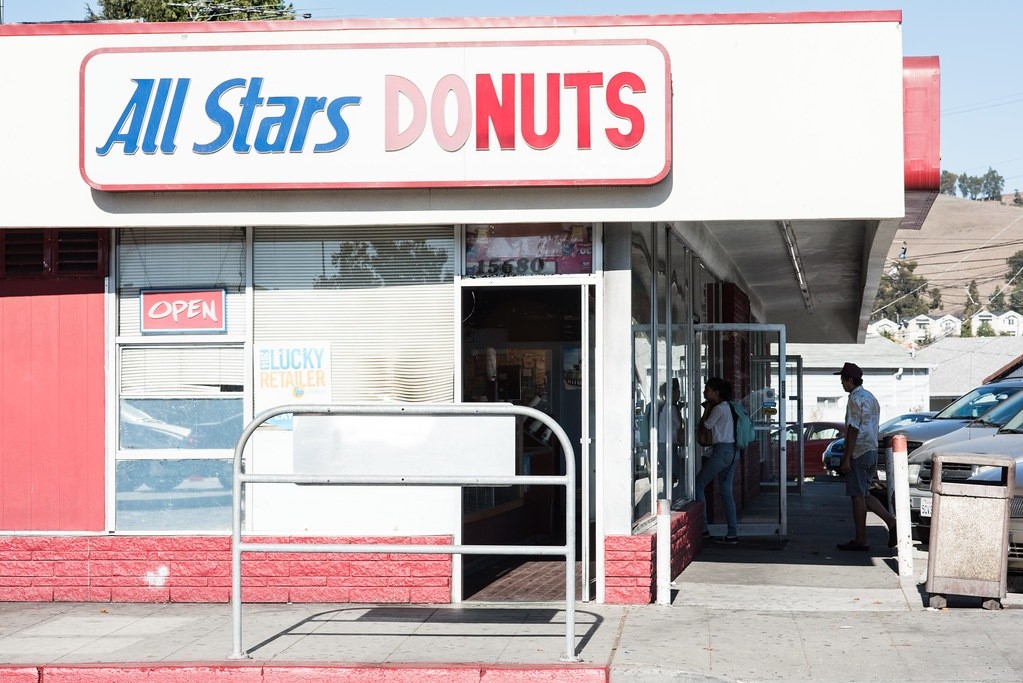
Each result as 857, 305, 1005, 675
832, 362, 863, 379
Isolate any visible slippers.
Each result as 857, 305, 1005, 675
887, 525, 897, 548
837, 539, 870, 551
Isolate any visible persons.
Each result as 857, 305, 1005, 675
658, 378, 685, 499
695, 377, 741, 544
833, 362, 897, 552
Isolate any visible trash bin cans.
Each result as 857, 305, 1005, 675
922, 452, 1018, 610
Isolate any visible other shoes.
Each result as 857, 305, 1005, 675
716, 536, 738, 544
702, 530, 709, 537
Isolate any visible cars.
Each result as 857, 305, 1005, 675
112, 398, 244, 491
822, 412, 939, 470
908, 389, 1023, 575
770, 421, 847, 482
883, 380, 1023, 464
